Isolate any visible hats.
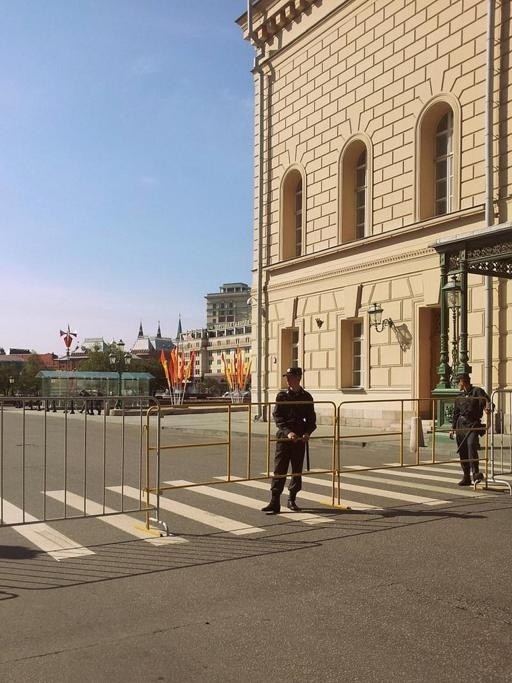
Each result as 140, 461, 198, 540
282, 367, 302, 377
453, 372, 470, 383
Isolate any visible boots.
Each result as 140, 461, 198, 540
261, 490, 281, 513
471, 461, 483, 483
287, 490, 300, 510
459, 467, 471, 486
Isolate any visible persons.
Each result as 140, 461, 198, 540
95, 388, 105, 414
80, 389, 90, 414
261, 366, 317, 514
448, 371, 496, 486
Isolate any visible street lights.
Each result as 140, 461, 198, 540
441, 274, 462, 389
108, 338, 132, 409
8, 376, 15, 397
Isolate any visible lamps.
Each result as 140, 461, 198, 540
367, 302, 404, 334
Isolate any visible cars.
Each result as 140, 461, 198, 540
222, 391, 250, 397
